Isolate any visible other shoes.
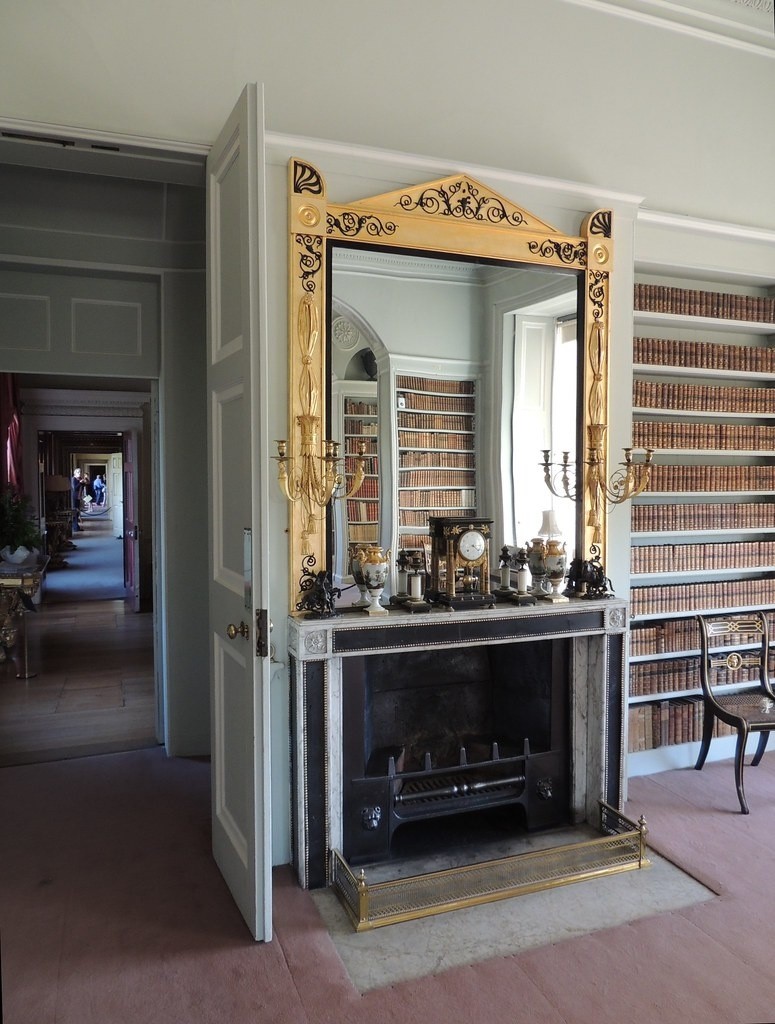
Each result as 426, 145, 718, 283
73, 528, 84, 532
85, 509, 94, 513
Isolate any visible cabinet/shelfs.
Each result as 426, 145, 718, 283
395, 373, 482, 555
339, 380, 381, 582
633, 265, 775, 753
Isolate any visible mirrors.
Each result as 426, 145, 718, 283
284, 157, 612, 614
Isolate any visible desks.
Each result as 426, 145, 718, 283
52, 508, 77, 550
45, 521, 69, 569
0, 554, 51, 679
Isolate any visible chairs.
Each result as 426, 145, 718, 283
693, 611, 775, 815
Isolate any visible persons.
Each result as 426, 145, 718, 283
71, 468, 84, 533
83, 473, 95, 514
94, 475, 104, 506
102, 474, 107, 506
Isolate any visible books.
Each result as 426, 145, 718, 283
630, 280, 775, 751
343, 372, 478, 580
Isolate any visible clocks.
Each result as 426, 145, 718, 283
427, 516, 497, 612
